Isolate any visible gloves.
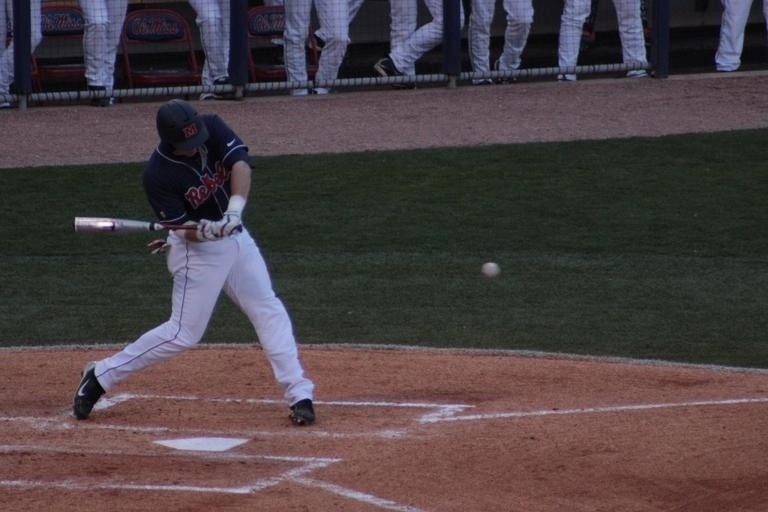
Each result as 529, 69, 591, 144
195, 219, 222, 243
218, 210, 243, 237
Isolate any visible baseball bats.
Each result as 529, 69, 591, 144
74, 217, 243, 235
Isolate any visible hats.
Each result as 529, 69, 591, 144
156, 98, 210, 149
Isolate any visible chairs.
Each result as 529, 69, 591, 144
1, 1, 319, 96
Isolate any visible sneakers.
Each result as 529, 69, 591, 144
214, 77, 232, 94
374, 57, 402, 88
73, 360, 104, 420
495, 59, 517, 85
289, 399, 316, 427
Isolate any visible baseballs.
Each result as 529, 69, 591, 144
482, 263, 500, 276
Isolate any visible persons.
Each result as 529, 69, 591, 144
466, 0, 535, 84
79, 0, 129, 106
373, 1, 466, 90
70, 98, 316, 424
714, 0, 768, 73
188, 1, 232, 101
0, 0, 42, 110
283, 0, 350, 96
556, 1, 647, 82
